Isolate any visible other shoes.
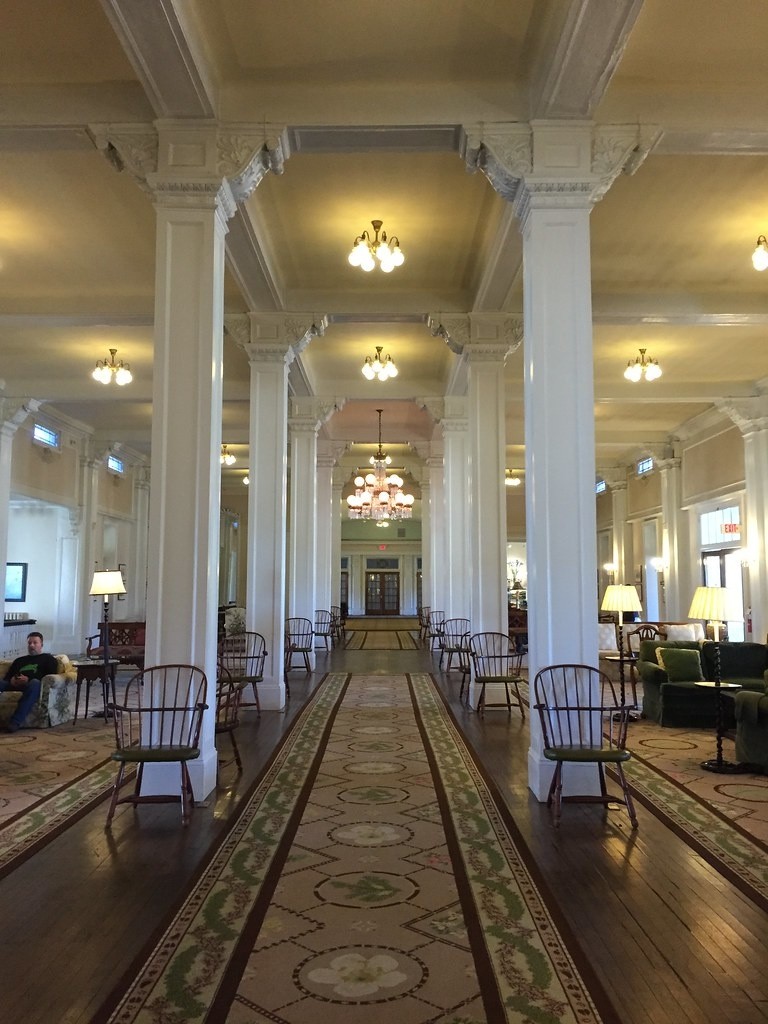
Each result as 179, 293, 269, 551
5, 719, 20, 732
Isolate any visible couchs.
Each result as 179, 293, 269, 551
598, 620, 768, 773
0, 654, 78, 729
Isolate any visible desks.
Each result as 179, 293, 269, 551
69, 660, 119, 726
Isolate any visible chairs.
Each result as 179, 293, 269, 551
418, 606, 527, 721
106, 664, 207, 827
216, 606, 346, 785
533, 664, 639, 831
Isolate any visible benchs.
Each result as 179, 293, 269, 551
85, 622, 145, 686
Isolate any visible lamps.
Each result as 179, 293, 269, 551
751, 235, 768, 271
623, 348, 662, 382
91, 348, 132, 385
347, 409, 414, 520
361, 346, 398, 381
689, 585, 738, 773
601, 585, 642, 722
348, 220, 405, 273
220, 445, 236, 465
88, 569, 127, 716
505, 469, 521, 486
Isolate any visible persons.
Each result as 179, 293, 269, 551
0, 632, 58, 733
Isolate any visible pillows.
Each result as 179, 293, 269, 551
656, 647, 705, 683
